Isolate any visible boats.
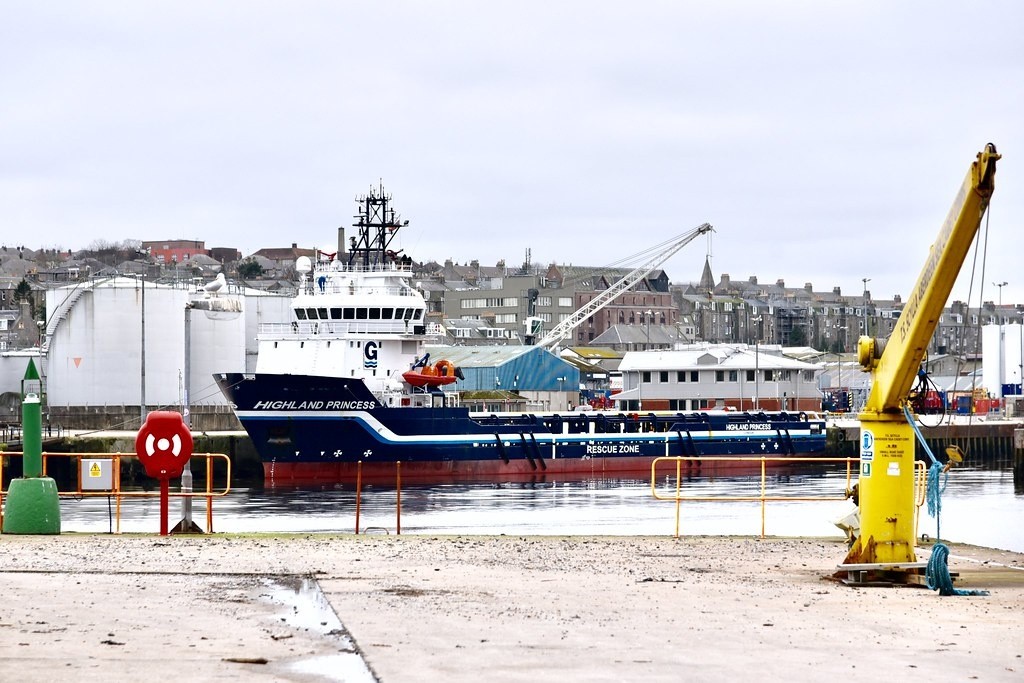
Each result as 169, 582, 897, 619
213, 181, 824, 493
